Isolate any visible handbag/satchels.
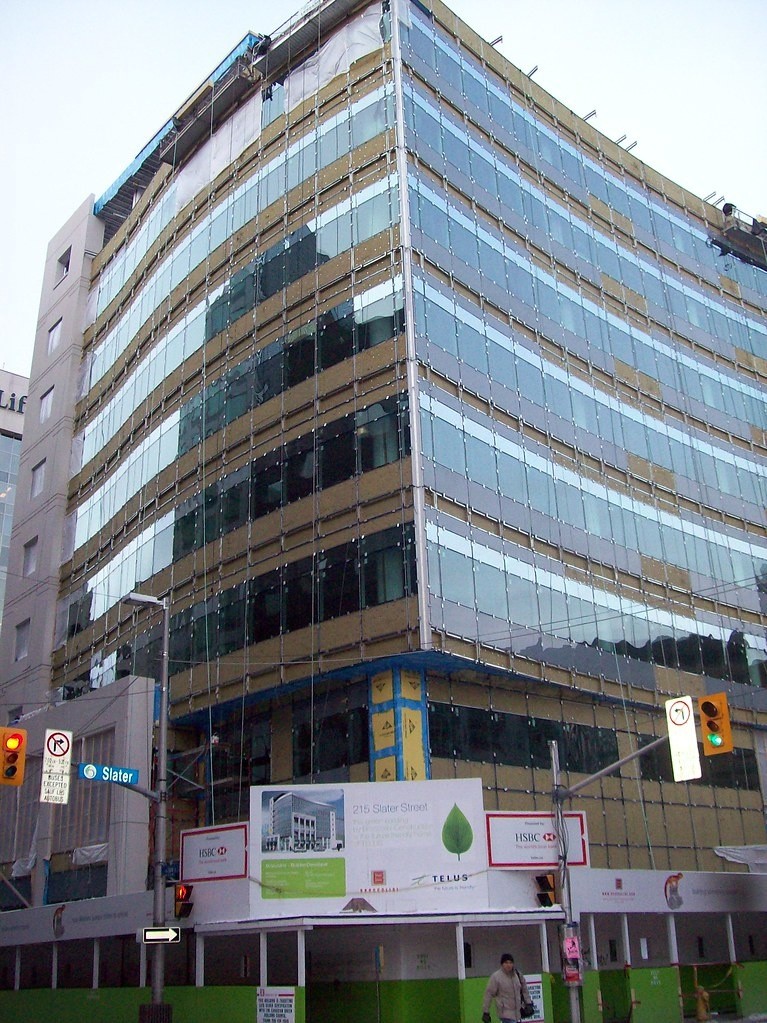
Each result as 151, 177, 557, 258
520, 1005, 534, 1018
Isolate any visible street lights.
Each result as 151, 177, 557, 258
120, 592, 172, 1005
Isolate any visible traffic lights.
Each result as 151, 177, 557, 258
0, 726, 27, 785
698, 692, 733, 757
174, 884, 194, 918
535, 874, 557, 907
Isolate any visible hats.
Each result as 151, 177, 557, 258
501, 954, 513, 965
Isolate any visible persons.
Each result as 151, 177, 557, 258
664, 872, 684, 910
481, 952, 531, 1023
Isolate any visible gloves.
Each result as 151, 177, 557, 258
482, 1012, 491, 1023
